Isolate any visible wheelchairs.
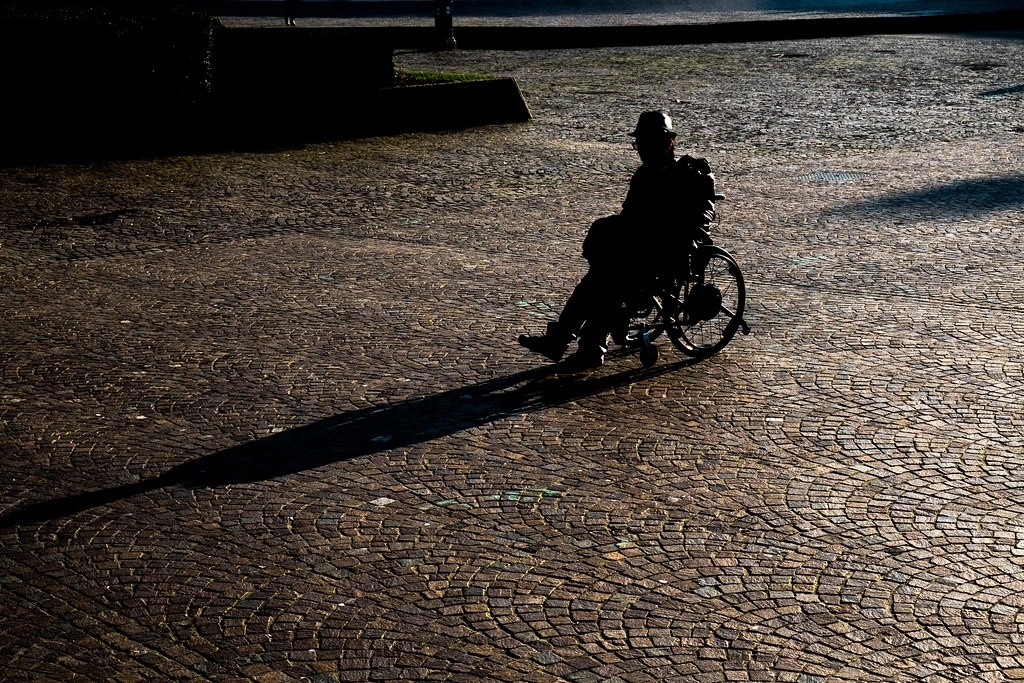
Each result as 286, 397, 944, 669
571, 194, 751, 368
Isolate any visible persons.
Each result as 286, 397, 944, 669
518, 108, 715, 374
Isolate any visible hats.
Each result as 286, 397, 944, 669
627, 111, 683, 137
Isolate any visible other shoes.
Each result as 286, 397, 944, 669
564, 340, 605, 366
517, 331, 571, 363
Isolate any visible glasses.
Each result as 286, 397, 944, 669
632, 141, 639, 150
